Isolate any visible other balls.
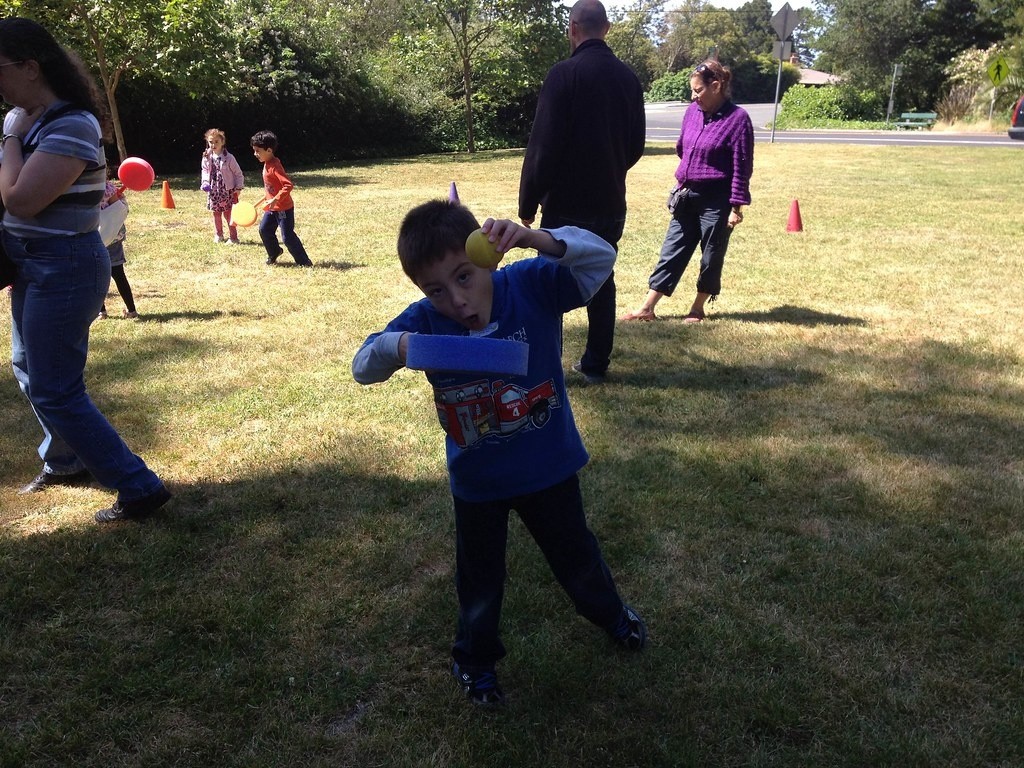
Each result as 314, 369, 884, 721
465, 230, 504, 268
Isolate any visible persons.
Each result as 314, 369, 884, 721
352, 203, 650, 712
199, 129, 245, 247
95, 158, 142, 319
517, 1, 646, 388
0, 14, 173, 524
617, 57, 754, 329
249, 131, 313, 266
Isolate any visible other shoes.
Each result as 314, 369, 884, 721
99, 313, 106, 319
215, 236, 224, 243
123, 310, 137, 318
227, 239, 240, 244
684, 311, 705, 323
266, 248, 283, 264
572, 360, 603, 383
621, 313, 657, 322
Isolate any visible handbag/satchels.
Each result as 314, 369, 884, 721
667, 188, 688, 213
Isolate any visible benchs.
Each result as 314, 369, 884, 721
893, 112, 937, 131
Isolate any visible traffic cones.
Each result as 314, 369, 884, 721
449, 182, 464, 206
784, 199, 805, 233
161, 181, 176, 209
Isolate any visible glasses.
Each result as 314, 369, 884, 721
693, 64, 718, 81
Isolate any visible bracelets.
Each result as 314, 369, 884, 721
2, 133, 19, 143
731, 204, 739, 213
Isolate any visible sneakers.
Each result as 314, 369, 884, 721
450, 662, 504, 709
94, 485, 172, 521
18, 470, 94, 495
609, 606, 645, 652
